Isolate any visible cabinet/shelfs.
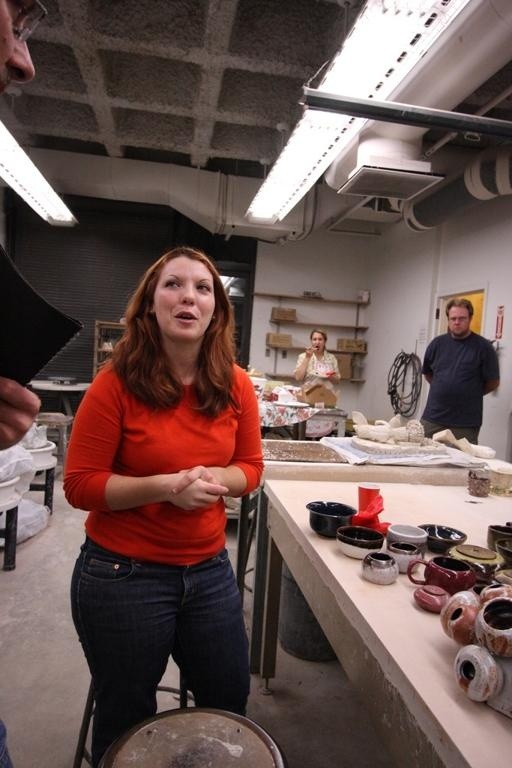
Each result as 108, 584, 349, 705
92, 320, 130, 380
252, 287, 371, 383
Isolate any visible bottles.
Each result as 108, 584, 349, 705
474, 596, 511, 660
361, 552, 400, 585
386, 541, 423, 574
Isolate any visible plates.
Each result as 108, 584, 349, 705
274, 401, 309, 408
311, 369, 330, 378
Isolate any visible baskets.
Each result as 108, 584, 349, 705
267, 333, 292, 347
272, 307, 297, 322
337, 338, 366, 354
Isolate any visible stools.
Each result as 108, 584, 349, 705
68, 642, 194, 767
36, 410, 76, 466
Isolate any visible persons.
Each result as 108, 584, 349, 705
419, 299, 500, 447
63, 246, 264, 766
1, 0, 41, 453
294, 328, 341, 394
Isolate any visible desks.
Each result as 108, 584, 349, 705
247, 478, 512, 765
231, 437, 512, 676
24, 439, 57, 517
24, 375, 92, 415
0, 477, 22, 574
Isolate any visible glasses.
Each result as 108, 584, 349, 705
13, 0, 48, 43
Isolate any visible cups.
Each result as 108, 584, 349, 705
468, 470, 492, 499
315, 402, 324, 409
249, 377, 267, 403
386, 524, 430, 559
358, 483, 381, 515
492, 469, 512, 496
406, 556, 476, 591
487, 524, 511, 562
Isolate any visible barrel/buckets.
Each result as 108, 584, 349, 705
279, 558, 336, 663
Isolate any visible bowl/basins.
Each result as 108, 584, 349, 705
23, 441, 57, 467
417, 523, 468, 554
336, 525, 385, 559
1, 476, 22, 505
306, 500, 358, 537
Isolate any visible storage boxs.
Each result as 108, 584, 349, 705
337, 336, 366, 353
308, 410, 347, 439
295, 383, 338, 408
265, 330, 294, 347
332, 353, 353, 380
271, 304, 296, 322
